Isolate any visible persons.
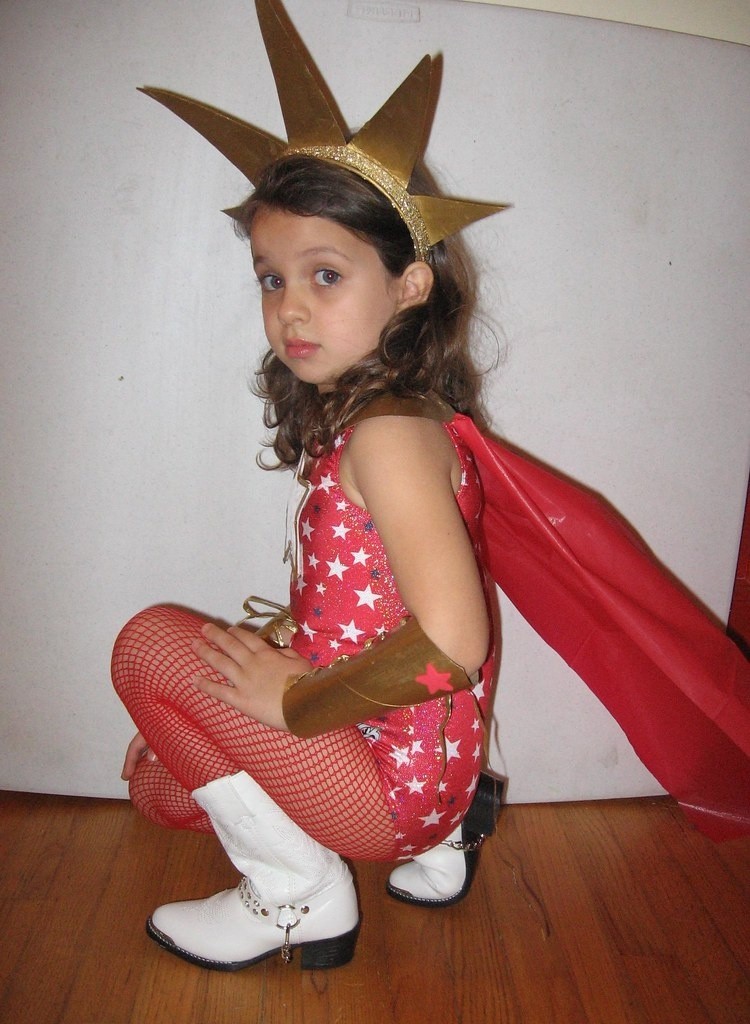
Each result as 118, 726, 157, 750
110, 153, 500, 973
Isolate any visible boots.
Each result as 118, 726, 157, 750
146, 769, 361, 970
387, 770, 505, 908
